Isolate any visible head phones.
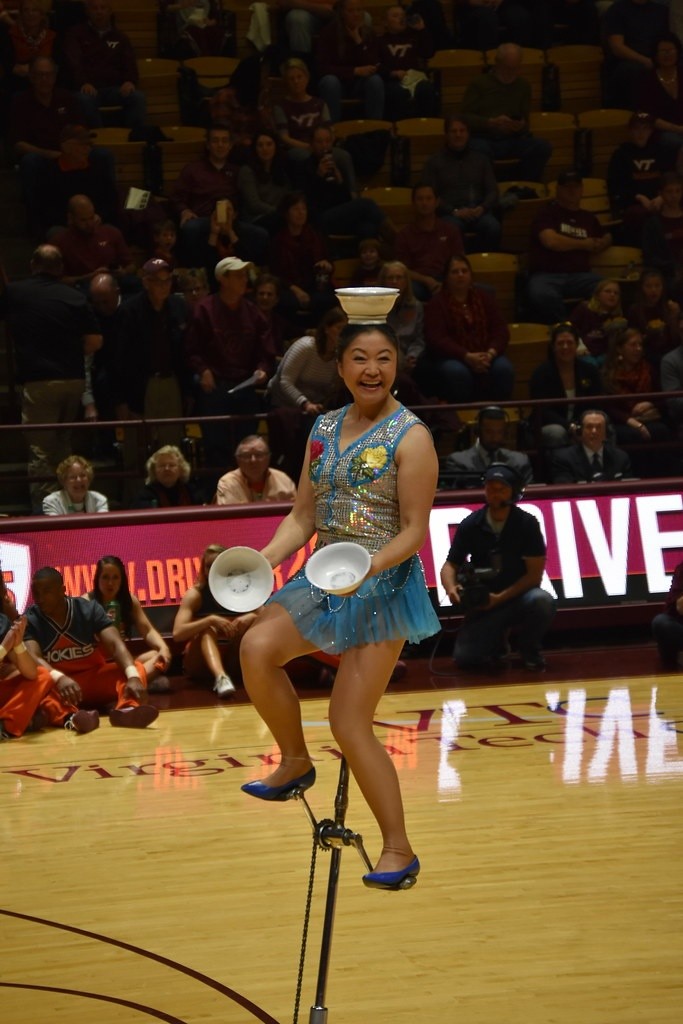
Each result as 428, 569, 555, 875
473, 406, 511, 438
481, 462, 527, 500
576, 422, 616, 439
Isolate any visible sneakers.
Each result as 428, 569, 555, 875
213, 673, 236, 697
109, 705, 160, 729
64, 711, 100, 734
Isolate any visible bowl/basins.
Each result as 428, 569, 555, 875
334, 286, 401, 295
334, 293, 399, 326
302, 541, 370, 594
207, 544, 276, 613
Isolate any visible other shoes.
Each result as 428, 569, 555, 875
521, 650, 545, 670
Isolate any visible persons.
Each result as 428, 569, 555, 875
238, 325, 442, 891
173, 545, 265, 696
440, 463, 556, 678
0, 0, 683, 733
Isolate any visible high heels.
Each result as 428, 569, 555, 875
361, 854, 420, 888
240, 766, 316, 802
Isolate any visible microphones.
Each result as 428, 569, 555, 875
500, 500, 507, 509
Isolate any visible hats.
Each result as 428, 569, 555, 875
483, 462, 519, 489
558, 171, 582, 190
215, 256, 250, 278
143, 259, 171, 276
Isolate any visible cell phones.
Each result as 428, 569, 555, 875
216, 201, 227, 223
325, 149, 333, 160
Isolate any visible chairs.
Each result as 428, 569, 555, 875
0, 0, 683, 480
